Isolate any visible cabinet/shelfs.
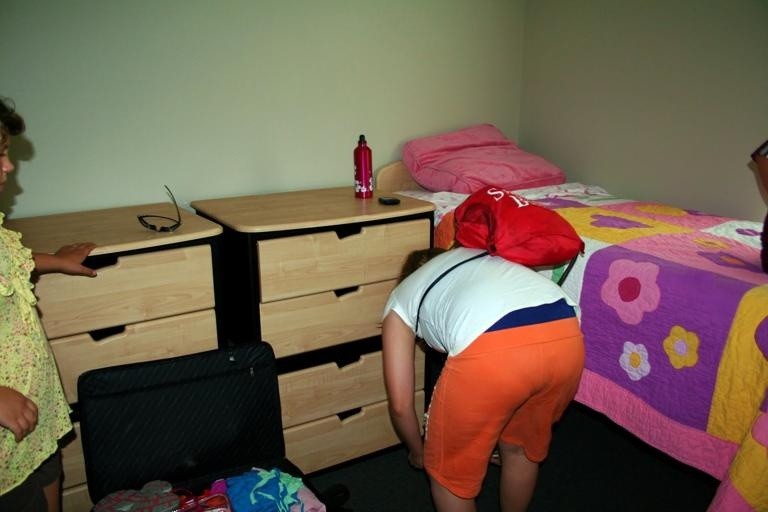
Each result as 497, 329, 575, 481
3, 202, 230, 512
186, 187, 437, 483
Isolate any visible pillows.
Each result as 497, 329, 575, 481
403, 122, 565, 197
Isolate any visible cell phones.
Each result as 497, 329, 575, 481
379, 197, 400, 205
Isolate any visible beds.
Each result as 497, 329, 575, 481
376, 156, 767, 511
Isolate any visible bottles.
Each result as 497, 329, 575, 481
351, 135, 377, 201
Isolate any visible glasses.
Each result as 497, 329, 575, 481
136, 184, 180, 232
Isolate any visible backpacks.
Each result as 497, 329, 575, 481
414, 183, 584, 335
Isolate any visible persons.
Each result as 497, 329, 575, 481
381, 239, 589, 510
0, 97, 99, 511
